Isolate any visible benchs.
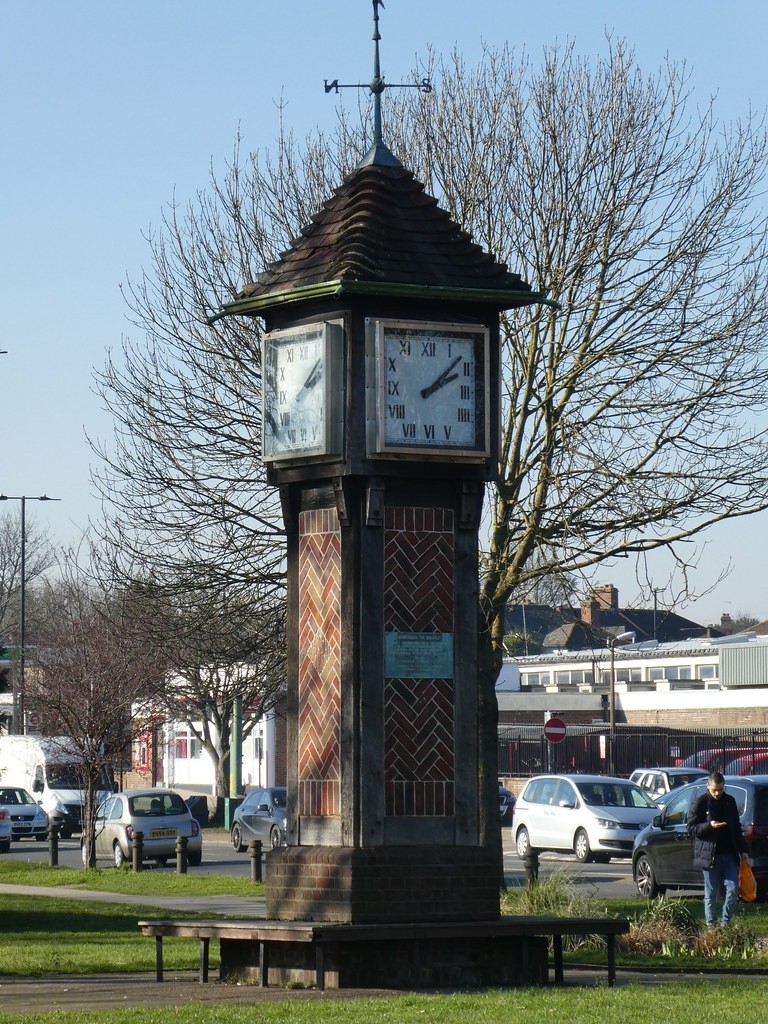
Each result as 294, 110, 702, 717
137, 916, 637, 991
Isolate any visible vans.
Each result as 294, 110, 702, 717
512, 746, 768, 903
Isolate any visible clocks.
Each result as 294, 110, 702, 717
375, 321, 490, 456
261, 320, 342, 464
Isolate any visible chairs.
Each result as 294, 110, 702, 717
151, 799, 163, 810
593, 794, 601, 803
606, 792, 617, 804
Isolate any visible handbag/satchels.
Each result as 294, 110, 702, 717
738, 858, 757, 901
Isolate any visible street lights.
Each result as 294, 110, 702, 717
607, 631, 636, 775
0, 493, 61, 732
654, 587, 666, 639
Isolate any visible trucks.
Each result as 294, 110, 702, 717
0, 735, 119, 839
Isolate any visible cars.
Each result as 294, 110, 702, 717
497, 782, 517, 826
81, 789, 202, 869
231, 788, 288, 853
0, 786, 49, 852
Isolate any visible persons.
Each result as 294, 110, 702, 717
671, 777, 686, 789
689, 772, 750, 932
273, 797, 284, 807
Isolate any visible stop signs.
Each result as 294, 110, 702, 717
545, 719, 566, 743
